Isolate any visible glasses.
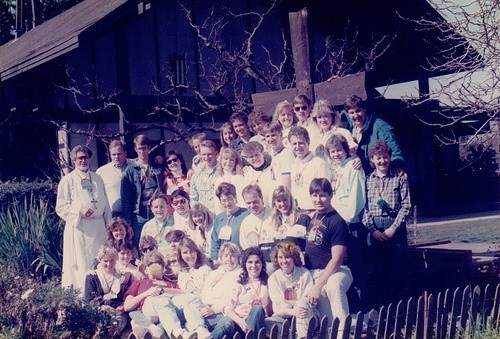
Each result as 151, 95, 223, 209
142, 245, 155, 253
147, 261, 161, 267
167, 157, 179, 166
78, 156, 89, 160
294, 105, 307, 111
172, 199, 186, 205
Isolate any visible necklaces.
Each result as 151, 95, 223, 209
74, 170, 98, 210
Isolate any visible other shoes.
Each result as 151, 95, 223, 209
174, 328, 197, 339
195, 326, 212, 339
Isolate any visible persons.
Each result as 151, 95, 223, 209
361, 142, 411, 311
200, 243, 244, 339
90, 217, 140, 270
151, 237, 213, 338
120, 134, 164, 257
326, 135, 366, 310
267, 242, 318, 339
303, 178, 357, 339
86, 242, 141, 280
309, 100, 362, 170
95, 140, 164, 217
123, 252, 211, 339
56, 145, 113, 326
338, 95, 405, 176
135, 94, 332, 296
84, 246, 130, 339
206, 246, 273, 339
